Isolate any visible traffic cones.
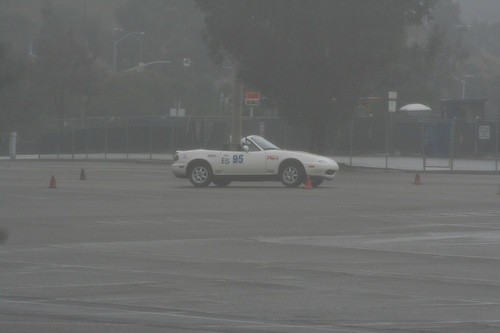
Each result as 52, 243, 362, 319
48, 176, 57, 188
79, 168, 87, 181
304, 176, 313, 188
413, 171, 422, 185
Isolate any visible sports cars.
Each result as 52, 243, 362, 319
170, 135, 339, 188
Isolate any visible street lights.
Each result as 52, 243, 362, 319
111, 31, 173, 79
